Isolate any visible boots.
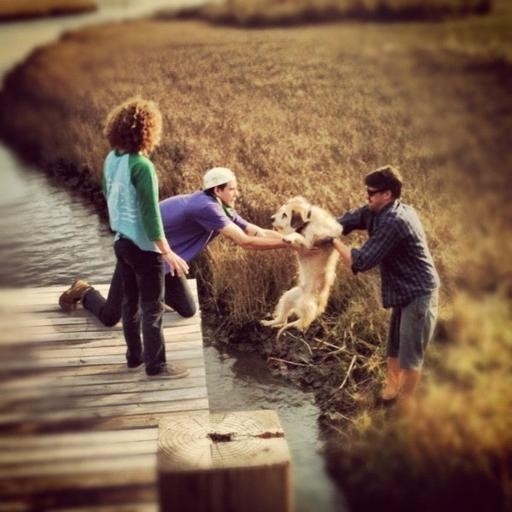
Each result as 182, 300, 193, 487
59, 280, 91, 313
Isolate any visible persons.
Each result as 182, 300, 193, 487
103, 97, 191, 380
59, 167, 320, 327
317, 165, 440, 408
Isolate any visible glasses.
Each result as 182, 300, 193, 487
367, 189, 384, 197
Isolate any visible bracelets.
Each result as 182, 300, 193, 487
161, 251, 171, 255
330, 237, 336, 245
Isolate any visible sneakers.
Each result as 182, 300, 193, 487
127, 360, 191, 380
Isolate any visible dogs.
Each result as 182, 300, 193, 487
256, 196, 344, 342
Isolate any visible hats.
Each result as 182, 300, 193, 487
365, 167, 403, 188
202, 167, 235, 193
